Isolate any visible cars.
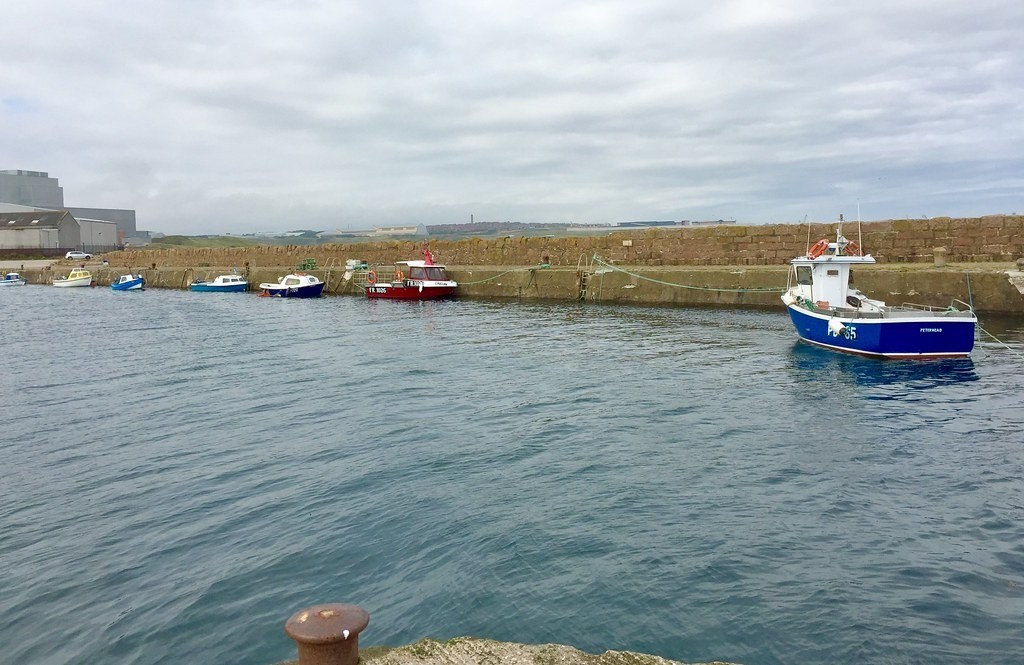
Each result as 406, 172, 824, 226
64, 250, 94, 261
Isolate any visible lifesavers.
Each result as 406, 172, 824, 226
808, 238, 829, 259
367, 271, 376, 283
296, 273, 306, 276
845, 239, 864, 257
847, 296, 861, 306
394, 271, 403, 281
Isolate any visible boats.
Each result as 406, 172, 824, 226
258, 273, 327, 299
110, 272, 145, 291
351, 234, 459, 300
0, 272, 28, 286
189, 266, 249, 293
780, 198, 979, 361
52, 267, 93, 287
